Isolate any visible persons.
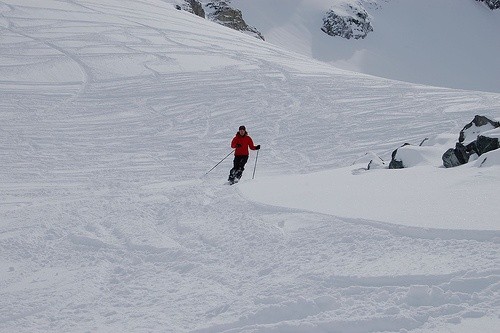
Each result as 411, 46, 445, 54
228, 125, 261, 185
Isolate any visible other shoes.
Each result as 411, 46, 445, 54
234, 176, 240, 181
228, 178, 234, 182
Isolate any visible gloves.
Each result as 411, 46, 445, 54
256, 145, 260, 150
236, 144, 241, 147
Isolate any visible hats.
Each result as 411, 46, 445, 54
239, 126, 245, 131
